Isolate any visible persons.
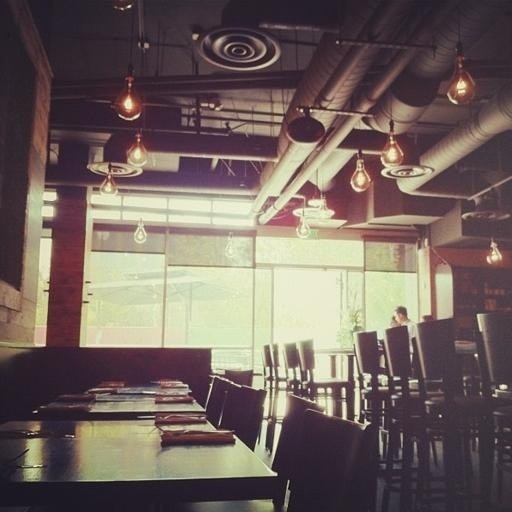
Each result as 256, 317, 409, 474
388, 306, 418, 352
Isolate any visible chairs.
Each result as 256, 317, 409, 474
260, 338, 356, 422
355, 310, 511, 511
208, 376, 380, 512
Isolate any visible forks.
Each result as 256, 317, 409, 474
156, 413, 213, 421
15, 429, 77, 439
171, 429, 233, 437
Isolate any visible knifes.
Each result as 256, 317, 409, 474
117, 390, 156, 395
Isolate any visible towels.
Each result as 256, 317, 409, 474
155, 412, 209, 424
48, 403, 92, 413
100, 381, 127, 387
155, 395, 194, 402
160, 429, 236, 448
58, 395, 97, 401
89, 388, 118, 393
161, 380, 183, 387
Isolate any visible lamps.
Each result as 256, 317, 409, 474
444, 18, 478, 107
296, 195, 312, 240
316, 170, 331, 220
126, 117, 148, 167
350, 123, 372, 193
222, 217, 237, 258
133, 197, 148, 244
379, 89, 405, 168
114, 8, 143, 121
99, 144, 119, 197
111, 1, 136, 11
484, 193, 501, 266
